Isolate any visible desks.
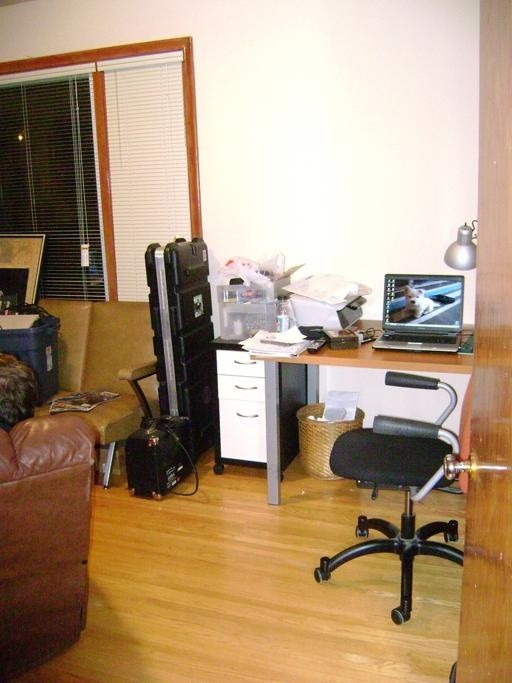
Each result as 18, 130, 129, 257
251, 320, 478, 505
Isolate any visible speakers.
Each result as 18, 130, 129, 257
124, 417, 194, 500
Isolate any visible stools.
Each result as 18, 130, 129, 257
315, 371, 463, 624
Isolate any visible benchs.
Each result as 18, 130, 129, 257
0, 296, 166, 489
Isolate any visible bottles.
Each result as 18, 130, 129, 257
275, 295, 290, 332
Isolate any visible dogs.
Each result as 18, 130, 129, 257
403, 285, 441, 319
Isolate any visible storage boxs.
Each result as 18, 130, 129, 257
213, 267, 290, 341
2, 307, 64, 404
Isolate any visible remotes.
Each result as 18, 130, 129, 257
307, 339, 327, 352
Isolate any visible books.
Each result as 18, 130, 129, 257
47, 391, 83, 405
48, 398, 78, 411
63, 389, 122, 411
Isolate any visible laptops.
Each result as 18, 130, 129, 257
371, 274, 464, 353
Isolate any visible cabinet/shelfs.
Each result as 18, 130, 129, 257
208, 342, 307, 469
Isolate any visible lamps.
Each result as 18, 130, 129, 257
444, 220, 479, 274
